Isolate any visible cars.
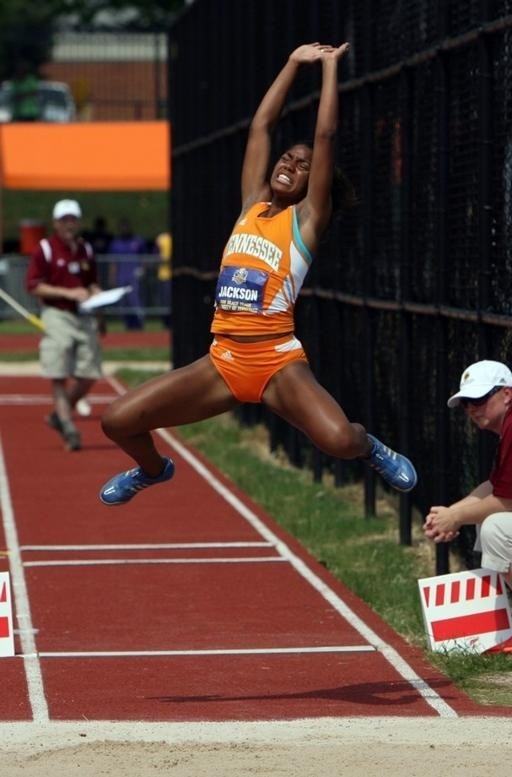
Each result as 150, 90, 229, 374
0, 77, 77, 123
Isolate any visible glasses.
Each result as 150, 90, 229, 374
461, 386, 503, 406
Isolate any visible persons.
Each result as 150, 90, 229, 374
423, 359, 512, 659
97, 37, 422, 508
5, 216, 174, 334
25, 196, 103, 453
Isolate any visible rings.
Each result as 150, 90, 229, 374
319, 49, 324, 53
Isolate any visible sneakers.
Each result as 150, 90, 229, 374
360, 433, 417, 492
45, 413, 81, 451
99, 455, 175, 506
75, 398, 91, 417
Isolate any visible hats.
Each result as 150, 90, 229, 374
447, 360, 512, 409
53, 200, 82, 219
